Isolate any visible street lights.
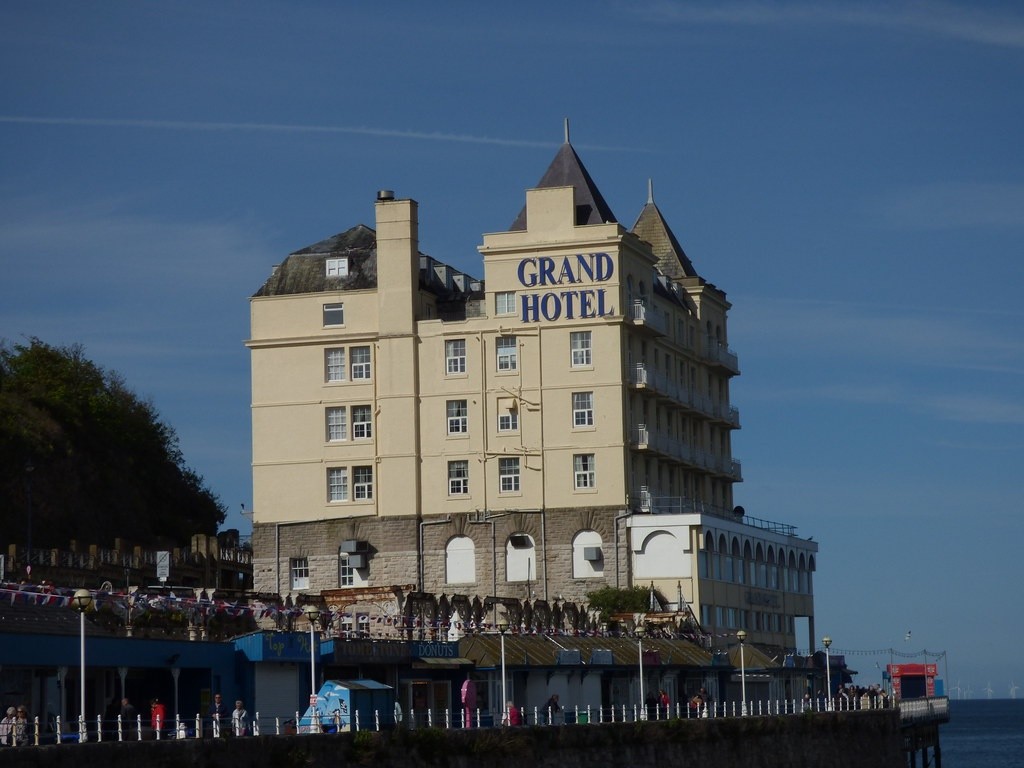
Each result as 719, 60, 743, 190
737, 631, 748, 716
496, 618, 510, 725
302, 606, 320, 734
68, 589, 94, 742
822, 637, 832, 711
633, 627, 648, 720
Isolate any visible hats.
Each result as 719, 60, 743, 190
151, 698, 159, 704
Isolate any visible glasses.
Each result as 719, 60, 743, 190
17, 710, 24, 713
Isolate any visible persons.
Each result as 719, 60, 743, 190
645, 689, 670, 719
151, 697, 166, 739
0, 705, 34, 746
232, 701, 251, 736
210, 693, 229, 736
503, 701, 521, 726
805, 683, 890, 710
17, 566, 40, 585
690, 688, 713, 717
121, 698, 137, 740
541, 695, 560, 724
